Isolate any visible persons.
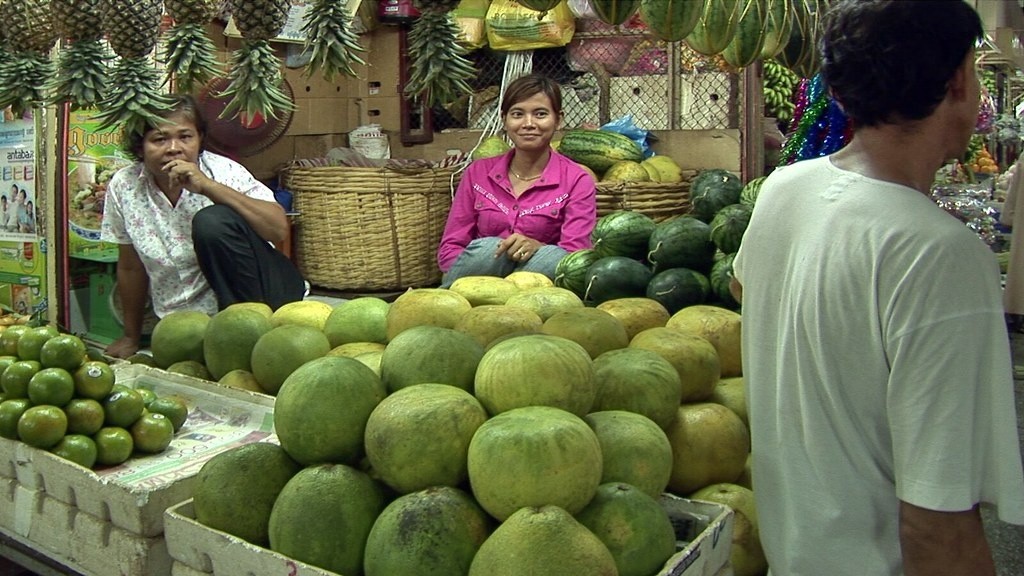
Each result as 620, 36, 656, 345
100, 93, 305, 360
998, 150, 1024, 334
0, 184, 35, 234
436, 73, 596, 290
733, 0, 1024, 576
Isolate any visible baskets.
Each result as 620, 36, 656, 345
589, 182, 691, 223
283, 155, 465, 290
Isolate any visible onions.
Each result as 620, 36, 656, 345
995, 164, 1016, 190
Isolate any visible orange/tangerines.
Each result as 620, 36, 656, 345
0, 324, 188, 471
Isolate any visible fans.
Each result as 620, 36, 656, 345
194, 70, 296, 187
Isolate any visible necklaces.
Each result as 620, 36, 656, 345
509, 164, 541, 181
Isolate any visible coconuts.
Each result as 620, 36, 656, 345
125, 272, 767, 576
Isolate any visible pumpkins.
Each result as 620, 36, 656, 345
971, 146, 998, 173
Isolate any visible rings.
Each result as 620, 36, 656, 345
519, 249, 523, 257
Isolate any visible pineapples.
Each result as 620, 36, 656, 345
0, 0, 370, 138
404, 0, 479, 106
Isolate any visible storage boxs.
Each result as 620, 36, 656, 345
162, 492, 735, 576
0, 363, 281, 576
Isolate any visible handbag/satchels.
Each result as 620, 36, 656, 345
485, 0, 576, 50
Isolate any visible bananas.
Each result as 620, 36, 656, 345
762, 59, 799, 119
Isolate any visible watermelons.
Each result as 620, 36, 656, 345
588, 0, 642, 24
469, 128, 684, 184
722, 0, 826, 79
555, 168, 768, 315
639, 0, 705, 41
516, 0, 561, 12
686, 0, 738, 55
